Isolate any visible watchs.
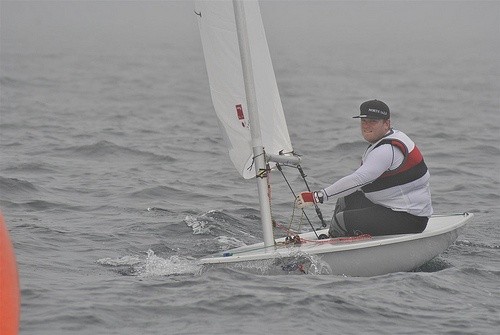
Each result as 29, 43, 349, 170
316, 190, 325, 203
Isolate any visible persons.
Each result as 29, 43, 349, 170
293, 99, 433, 239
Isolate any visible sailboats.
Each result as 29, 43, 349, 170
189, 0, 477, 273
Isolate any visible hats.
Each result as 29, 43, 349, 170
352, 98, 391, 119
